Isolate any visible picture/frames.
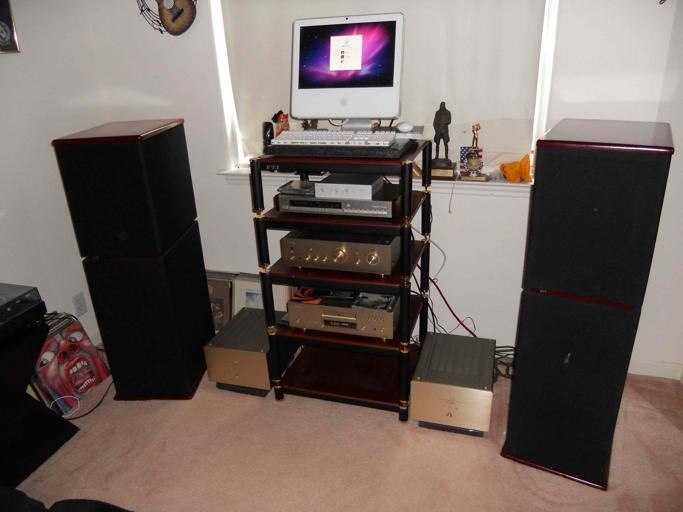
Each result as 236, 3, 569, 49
207, 273, 293, 332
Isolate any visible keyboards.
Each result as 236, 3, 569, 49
271, 130, 396, 147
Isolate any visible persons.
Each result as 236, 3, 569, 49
34, 322, 110, 414
433, 102, 451, 160
472, 124, 481, 149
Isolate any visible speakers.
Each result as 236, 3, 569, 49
51, 119, 197, 259
522, 117, 675, 308
82, 220, 214, 401
500, 290, 641, 491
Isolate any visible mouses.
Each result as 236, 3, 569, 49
396, 121, 413, 132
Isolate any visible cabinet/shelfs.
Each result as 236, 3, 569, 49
202, 305, 288, 399
248, 139, 434, 423
409, 328, 497, 436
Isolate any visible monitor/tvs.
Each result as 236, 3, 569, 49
290, 12, 403, 132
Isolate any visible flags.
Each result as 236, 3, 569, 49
461, 146, 482, 176
